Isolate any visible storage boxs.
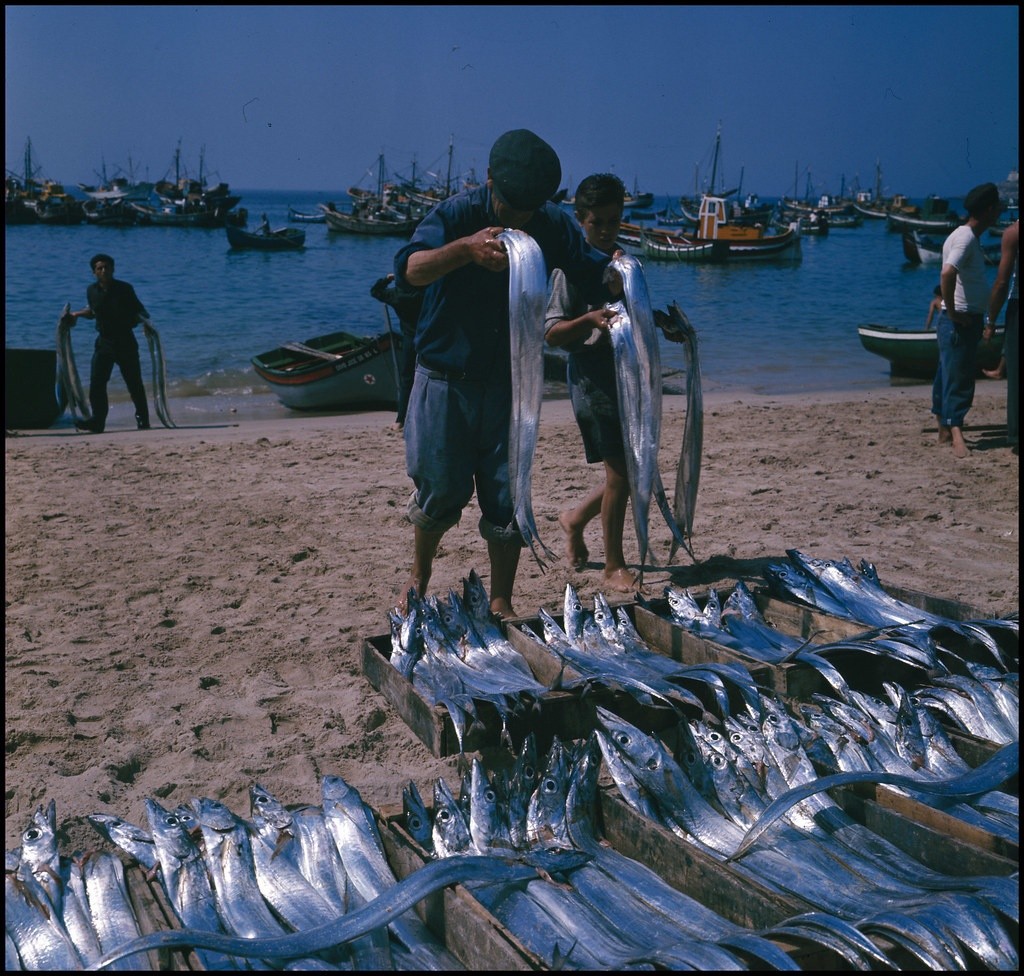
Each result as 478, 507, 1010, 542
362, 611, 593, 761
753, 579, 1020, 680
501, 601, 769, 744
634, 590, 908, 712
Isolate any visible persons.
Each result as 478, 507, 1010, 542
926, 283, 943, 333
68, 255, 158, 432
979, 217, 1020, 446
981, 338, 1008, 380
542, 173, 686, 596
391, 126, 629, 621
928, 181, 1007, 459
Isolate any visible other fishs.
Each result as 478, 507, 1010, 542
53, 301, 177, 434
5, 550, 1019, 971
494, 226, 703, 576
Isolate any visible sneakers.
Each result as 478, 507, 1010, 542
134, 410, 152, 432
73, 416, 105, 434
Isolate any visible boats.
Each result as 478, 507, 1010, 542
316, 131, 482, 236
542, 339, 571, 377
902, 231, 1002, 267
884, 193, 970, 235
638, 220, 730, 264
224, 223, 305, 249
251, 330, 405, 410
287, 203, 327, 223
857, 323, 1007, 372
3, 347, 76, 428
1006, 197, 1019, 211
771, 215, 830, 236
562, 163, 778, 232
988, 219, 1017, 235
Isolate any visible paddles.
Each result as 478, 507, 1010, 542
278, 340, 343, 361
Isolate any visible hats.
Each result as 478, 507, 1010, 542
489, 128, 563, 211
963, 183, 1002, 215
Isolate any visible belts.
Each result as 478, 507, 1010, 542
414, 352, 515, 385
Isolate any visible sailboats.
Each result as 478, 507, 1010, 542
781, 166, 854, 216
850, 157, 892, 219
5, 136, 248, 226
778, 160, 864, 228
616, 118, 795, 259
